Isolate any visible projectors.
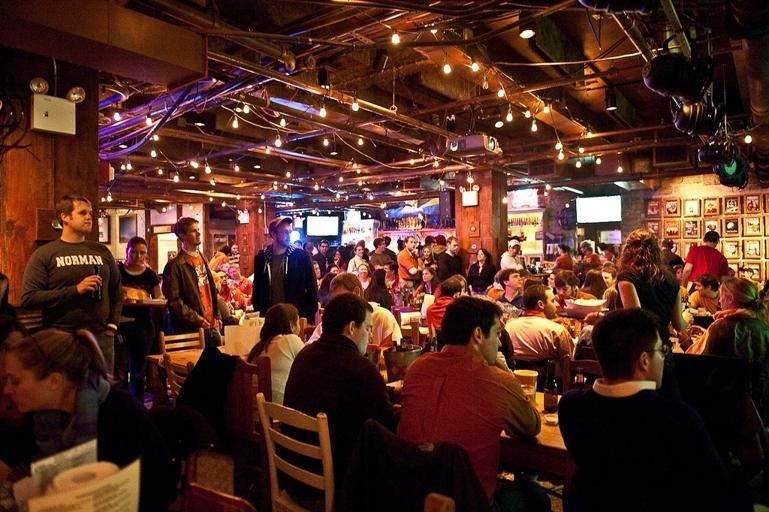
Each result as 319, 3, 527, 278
448, 136, 503, 156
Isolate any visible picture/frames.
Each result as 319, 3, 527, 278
642, 191, 769, 290
98, 215, 111, 245
118, 212, 138, 244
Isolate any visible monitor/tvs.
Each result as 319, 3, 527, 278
573, 193, 623, 226
292, 213, 305, 229
304, 213, 342, 240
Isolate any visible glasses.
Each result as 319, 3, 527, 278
644, 344, 670, 355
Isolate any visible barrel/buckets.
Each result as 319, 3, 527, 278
382, 344, 423, 383
365, 344, 381, 374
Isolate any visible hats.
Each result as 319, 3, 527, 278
269, 216, 292, 235
356, 259, 369, 272
705, 231, 719, 240
662, 238, 673, 245
508, 239, 521, 250
432, 235, 446, 245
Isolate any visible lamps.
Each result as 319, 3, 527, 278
513, 5, 537, 41
643, 40, 752, 191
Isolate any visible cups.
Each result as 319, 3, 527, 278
125, 297, 134, 303
698, 308, 705, 316
243, 318, 264, 326
513, 369, 539, 403
136, 299, 146, 303
544, 412, 559, 426
151, 299, 163, 304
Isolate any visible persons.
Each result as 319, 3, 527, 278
161, 215, 223, 346
5, 329, 161, 512
206, 215, 769, 512
113, 236, 166, 404
21, 192, 123, 380
0, 330, 41, 471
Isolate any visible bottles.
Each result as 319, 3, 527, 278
396, 291, 424, 308
574, 367, 585, 389
390, 335, 414, 352
91, 264, 104, 302
383, 212, 453, 230
543, 359, 558, 414
681, 304, 695, 334
508, 216, 540, 226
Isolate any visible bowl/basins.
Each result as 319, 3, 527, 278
563, 297, 608, 314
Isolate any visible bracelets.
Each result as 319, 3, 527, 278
105, 323, 119, 330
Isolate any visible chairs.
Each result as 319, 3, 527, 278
0, 296, 625, 487
255, 393, 335, 512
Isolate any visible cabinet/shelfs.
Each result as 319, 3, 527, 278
374, 191, 576, 265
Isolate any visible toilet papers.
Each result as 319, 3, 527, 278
45, 461, 119, 497
